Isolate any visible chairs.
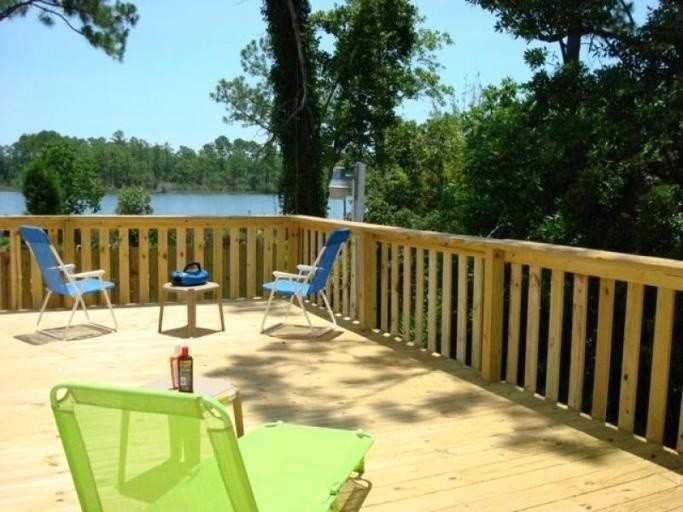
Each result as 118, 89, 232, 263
18, 224, 116, 341
51, 383, 375, 512
259, 228, 351, 340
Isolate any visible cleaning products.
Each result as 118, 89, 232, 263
178, 346, 193, 392
170, 346, 178, 389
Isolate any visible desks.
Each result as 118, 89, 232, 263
159, 280, 224, 337
117, 378, 243, 503
